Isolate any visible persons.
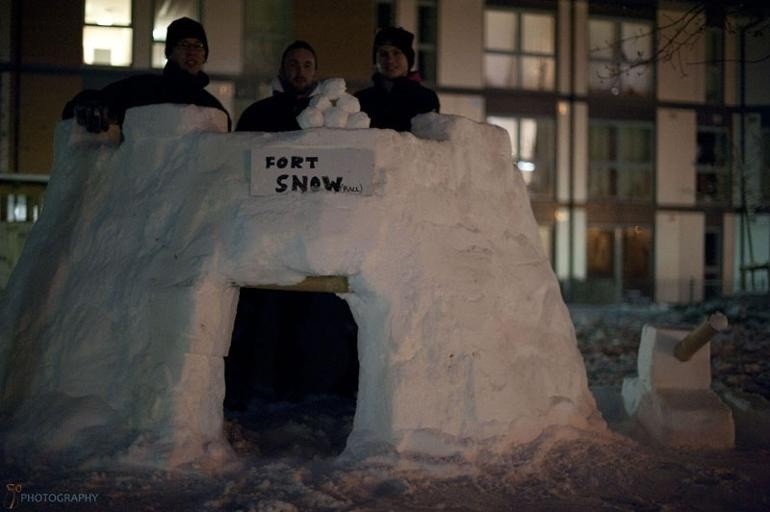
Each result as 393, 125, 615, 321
355, 25, 443, 132
232, 40, 324, 134
59, 16, 232, 137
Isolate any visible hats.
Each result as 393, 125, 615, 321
165, 17, 209, 60
371, 25, 416, 70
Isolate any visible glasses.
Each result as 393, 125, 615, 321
170, 40, 206, 52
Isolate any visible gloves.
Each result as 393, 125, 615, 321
72, 100, 119, 134
